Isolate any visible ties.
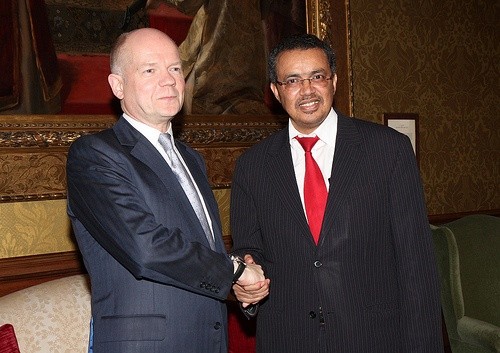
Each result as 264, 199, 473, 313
158, 133, 216, 253
294, 135, 328, 246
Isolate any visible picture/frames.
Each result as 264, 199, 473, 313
0, 0, 354, 204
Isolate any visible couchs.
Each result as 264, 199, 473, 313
0, 272, 93, 353
425, 213, 500, 353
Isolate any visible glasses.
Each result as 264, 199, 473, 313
277, 72, 334, 91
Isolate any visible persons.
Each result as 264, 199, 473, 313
65, 27, 265, 353
229, 33, 444, 352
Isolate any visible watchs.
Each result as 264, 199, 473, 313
233, 255, 247, 284
